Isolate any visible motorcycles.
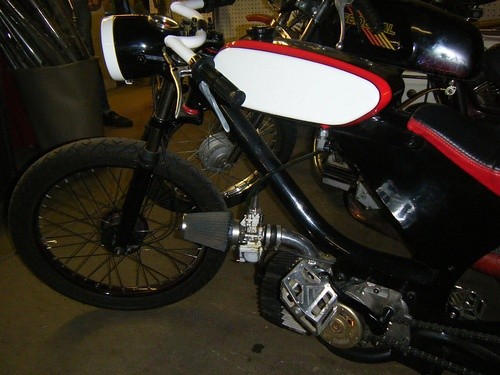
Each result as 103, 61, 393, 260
7, 0, 500, 375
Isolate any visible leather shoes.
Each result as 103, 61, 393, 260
102, 110, 134, 128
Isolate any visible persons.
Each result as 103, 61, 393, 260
68, 0, 133, 127
